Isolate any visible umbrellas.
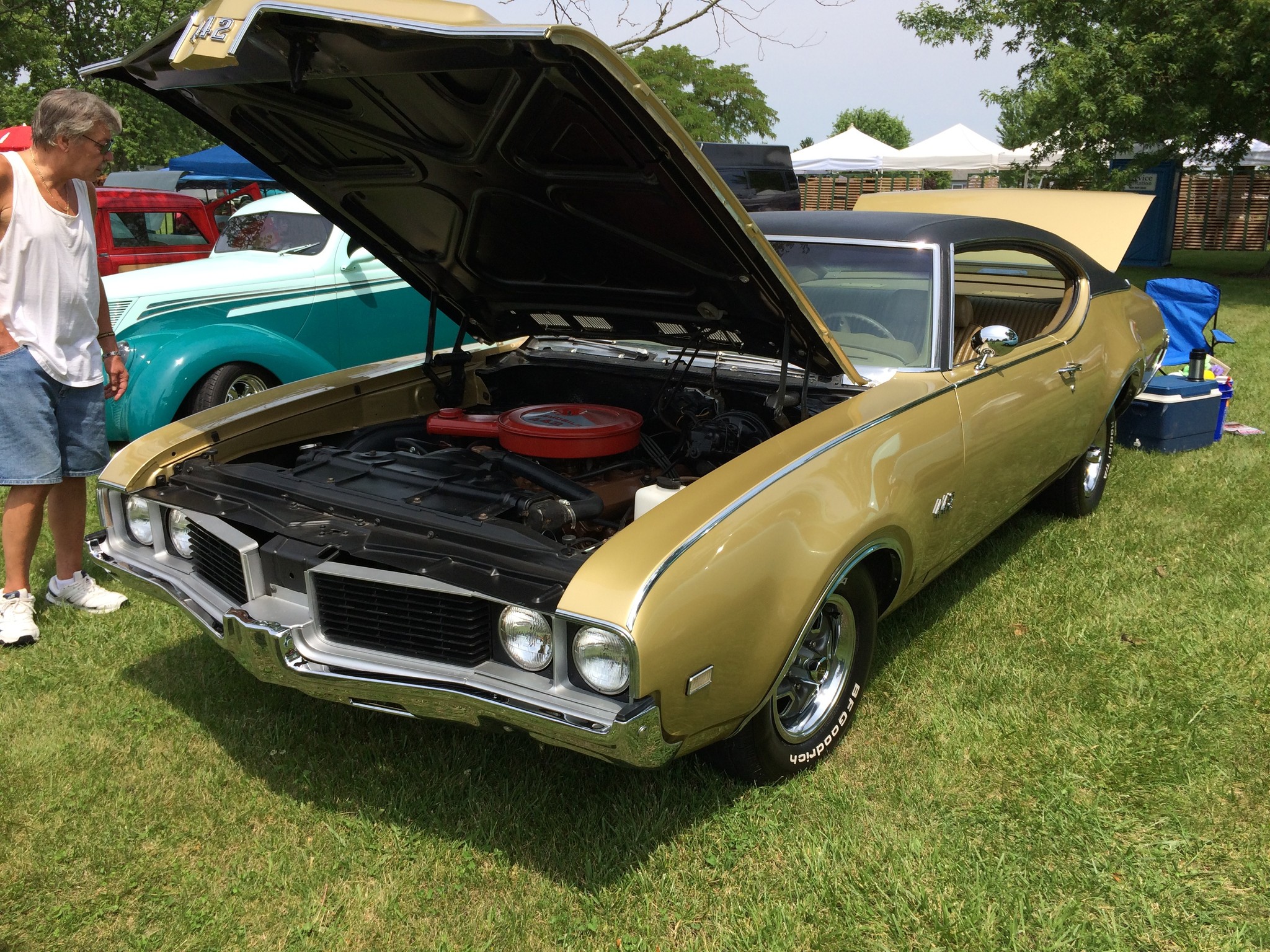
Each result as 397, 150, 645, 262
0, 124, 33, 151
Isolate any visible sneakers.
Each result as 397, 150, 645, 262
0, 588, 40, 648
46, 570, 130, 614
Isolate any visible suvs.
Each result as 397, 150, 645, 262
697, 140, 802, 215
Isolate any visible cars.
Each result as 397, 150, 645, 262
78, 0, 1169, 782
102, 143, 293, 248
77, 185, 469, 464
0, 123, 266, 274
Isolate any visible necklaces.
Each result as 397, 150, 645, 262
30, 146, 70, 216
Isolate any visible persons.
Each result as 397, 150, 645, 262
0, 88, 130, 645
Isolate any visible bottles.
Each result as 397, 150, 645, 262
1185, 348, 1207, 381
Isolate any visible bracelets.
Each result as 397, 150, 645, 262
97, 331, 116, 340
101, 350, 122, 362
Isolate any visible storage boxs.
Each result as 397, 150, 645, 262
1114, 375, 1222, 454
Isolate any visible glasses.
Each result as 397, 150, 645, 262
82, 135, 114, 154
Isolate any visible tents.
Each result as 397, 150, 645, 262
156, 145, 284, 235
788, 120, 1270, 177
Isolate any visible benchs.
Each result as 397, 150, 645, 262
800, 286, 1061, 364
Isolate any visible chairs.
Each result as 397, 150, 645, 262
1144, 277, 1236, 376
880, 289, 983, 365
